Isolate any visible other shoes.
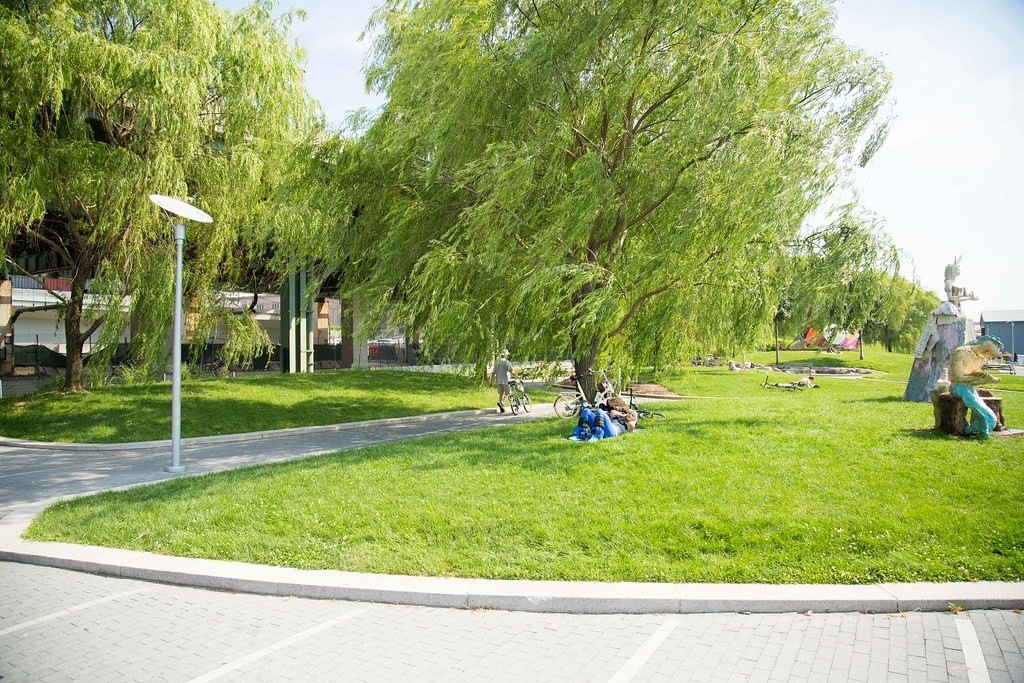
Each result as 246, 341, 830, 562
588, 436, 597, 442
569, 436, 582, 441
501, 409, 504, 413
497, 402, 503, 408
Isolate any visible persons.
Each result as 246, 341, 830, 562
989, 350, 1018, 364
943, 253, 980, 310
489, 348, 522, 413
569, 408, 637, 443
951, 334, 1004, 439
797, 376, 814, 388
211, 353, 227, 377
827, 347, 836, 353
729, 362, 745, 372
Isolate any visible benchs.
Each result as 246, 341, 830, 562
982, 367, 1016, 375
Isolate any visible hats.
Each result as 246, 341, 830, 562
500, 349, 509, 355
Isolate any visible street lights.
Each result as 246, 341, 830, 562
150, 194, 214, 474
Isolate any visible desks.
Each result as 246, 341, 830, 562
985, 364, 1012, 375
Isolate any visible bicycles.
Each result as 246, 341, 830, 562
760, 376, 804, 392
628, 386, 666, 422
507, 376, 532, 415
552, 360, 619, 420
